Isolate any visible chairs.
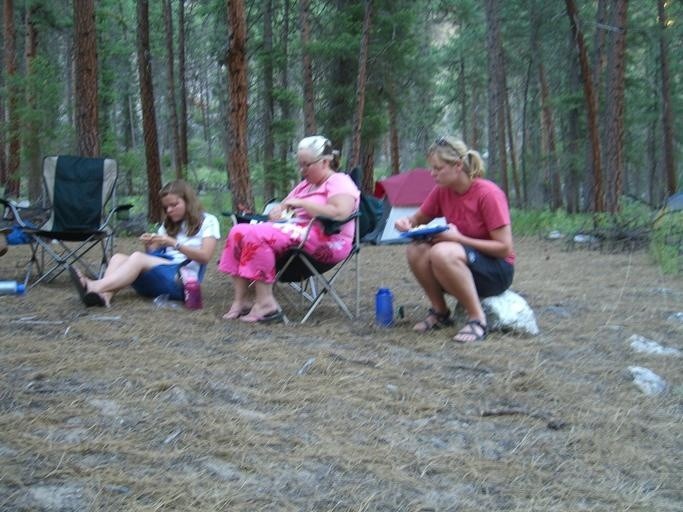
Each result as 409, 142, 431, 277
223, 166, 365, 324
0, 154, 119, 297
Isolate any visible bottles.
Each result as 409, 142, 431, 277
0, 280, 24, 296
376, 288, 394, 328
184, 277, 201, 309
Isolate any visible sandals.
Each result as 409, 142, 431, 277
413, 308, 451, 332
452, 320, 489, 343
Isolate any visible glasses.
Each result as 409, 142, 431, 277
436, 137, 459, 154
300, 160, 321, 170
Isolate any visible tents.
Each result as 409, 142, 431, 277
373, 170, 448, 245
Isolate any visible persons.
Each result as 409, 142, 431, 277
393, 136, 514, 343
70, 180, 221, 306
218, 135, 361, 324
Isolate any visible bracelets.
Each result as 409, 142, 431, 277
174, 241, 182, 250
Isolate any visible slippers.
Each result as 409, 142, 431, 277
70, 267, 110, 307
222, 306, 284, 322
